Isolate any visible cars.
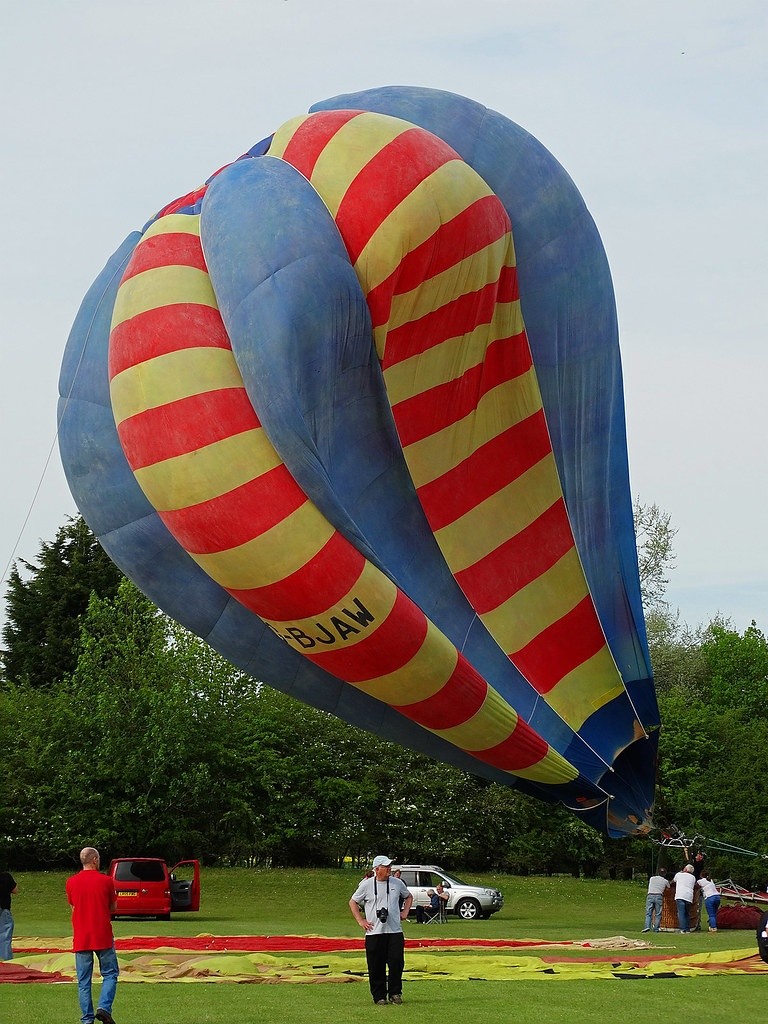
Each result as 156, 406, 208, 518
107, 858, 200, 920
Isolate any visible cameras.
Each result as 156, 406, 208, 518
377, 907, 389, 923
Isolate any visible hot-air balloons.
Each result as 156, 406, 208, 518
56, 86, 702, 932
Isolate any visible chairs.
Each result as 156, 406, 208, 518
423, 897, 448, 924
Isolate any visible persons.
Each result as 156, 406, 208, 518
0, 870, 20, 962
358, 869, 449, 923
66, 847, 119, 1024
349, 856, 413, 1004
642, 852, 720, 933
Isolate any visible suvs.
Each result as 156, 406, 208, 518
358, 865, 504, 919
